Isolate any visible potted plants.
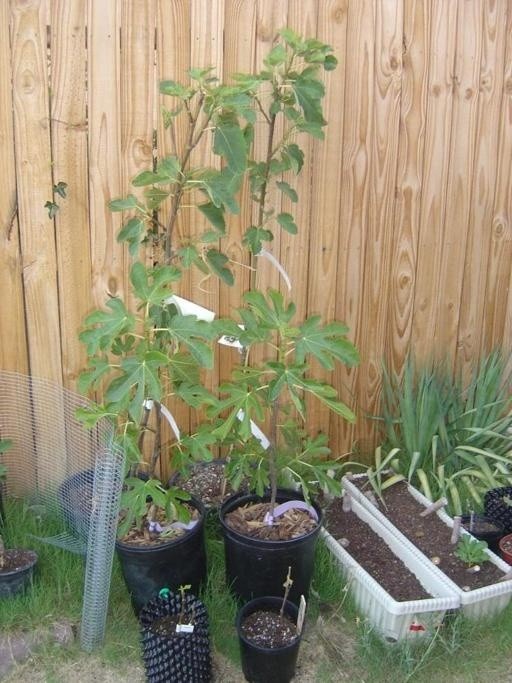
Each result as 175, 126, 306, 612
60, 472, 147, 549
302, 470, 460, 648
164, 456, 245, 534
0, 436, 40, 598
80, 65, 260, 625
235, 567, 307, 682
142, 584, 213, 681
497, 525, 511, 563
218, 32, 355, 608
342, 454, 512, 628
